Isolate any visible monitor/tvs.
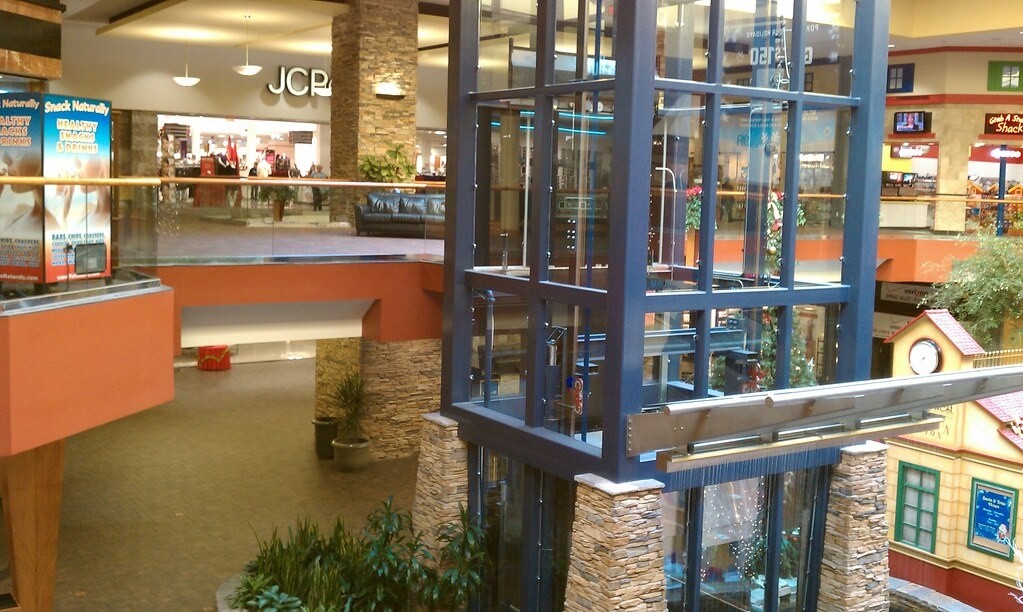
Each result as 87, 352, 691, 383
894, 111, 926, 134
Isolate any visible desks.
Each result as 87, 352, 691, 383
198, 344, 231, 371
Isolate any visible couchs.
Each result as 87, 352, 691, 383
352, 189, 446, 239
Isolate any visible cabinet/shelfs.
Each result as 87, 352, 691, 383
877, 201, 931, 230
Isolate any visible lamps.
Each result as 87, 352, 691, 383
313, 44, 335, 98
170, 32, 202, 89
375, 81, 409, 102
231, 14, 263, 77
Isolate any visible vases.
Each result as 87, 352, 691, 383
313, 414, 337, 457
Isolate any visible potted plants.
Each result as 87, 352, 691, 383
324, 368, 378, 474
258, 185, 298, 222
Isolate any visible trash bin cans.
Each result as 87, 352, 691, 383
312, 416, 339, 459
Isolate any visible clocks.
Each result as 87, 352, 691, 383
908, 337, 942, 375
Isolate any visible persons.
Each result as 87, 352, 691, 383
904, 113, 919, 131
0, 149, 42, 237
160, 135, 172, 202
290, 164, 300, 203
257, 156, 272, 177
218, 150, 230, 175
720, 177, 735, 222
249, 162, 259, 201
304, 164, 328, 211
56, 158, 111, 230
